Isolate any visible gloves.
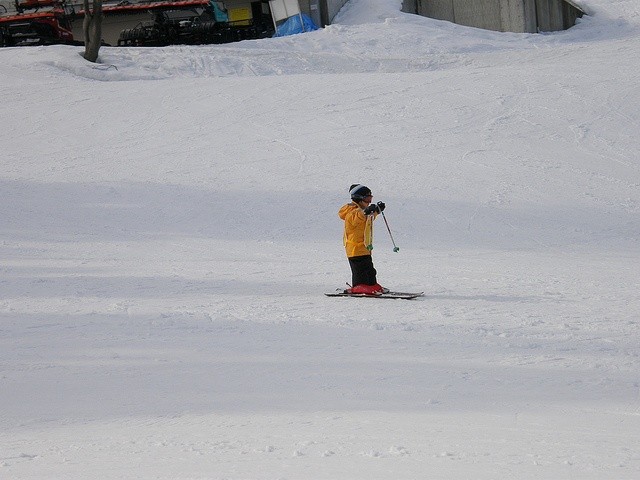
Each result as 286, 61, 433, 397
377, 201, 385, 211
364, 204, 381, 216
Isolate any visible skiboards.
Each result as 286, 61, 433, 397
325, 290, 424, 299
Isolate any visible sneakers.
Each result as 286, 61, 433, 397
347, 285, 382, 295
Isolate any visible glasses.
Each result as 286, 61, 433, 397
352, 194, 373, 203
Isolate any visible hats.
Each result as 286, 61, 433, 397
349, 184, 371, 202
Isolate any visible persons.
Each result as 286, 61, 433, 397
338, 184, 389, 296
